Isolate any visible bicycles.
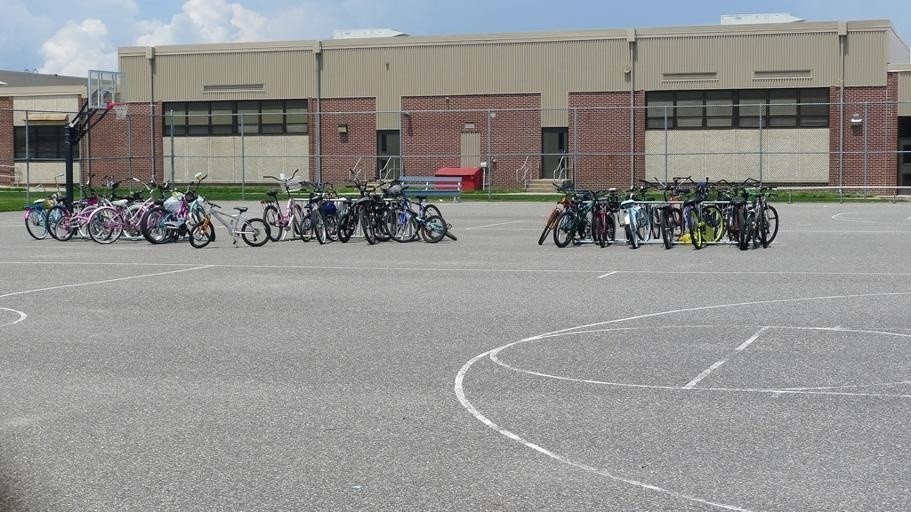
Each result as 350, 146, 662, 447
534, 173, 781, 254
17, 171, 216, 245
296, 164, 459, 243
187, 199, 271, 250
256, 167, 309, 242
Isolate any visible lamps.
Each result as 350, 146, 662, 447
338, 123, 348, 135
850, 112, 863, 127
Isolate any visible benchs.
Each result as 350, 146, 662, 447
398, 175, 463, 205
